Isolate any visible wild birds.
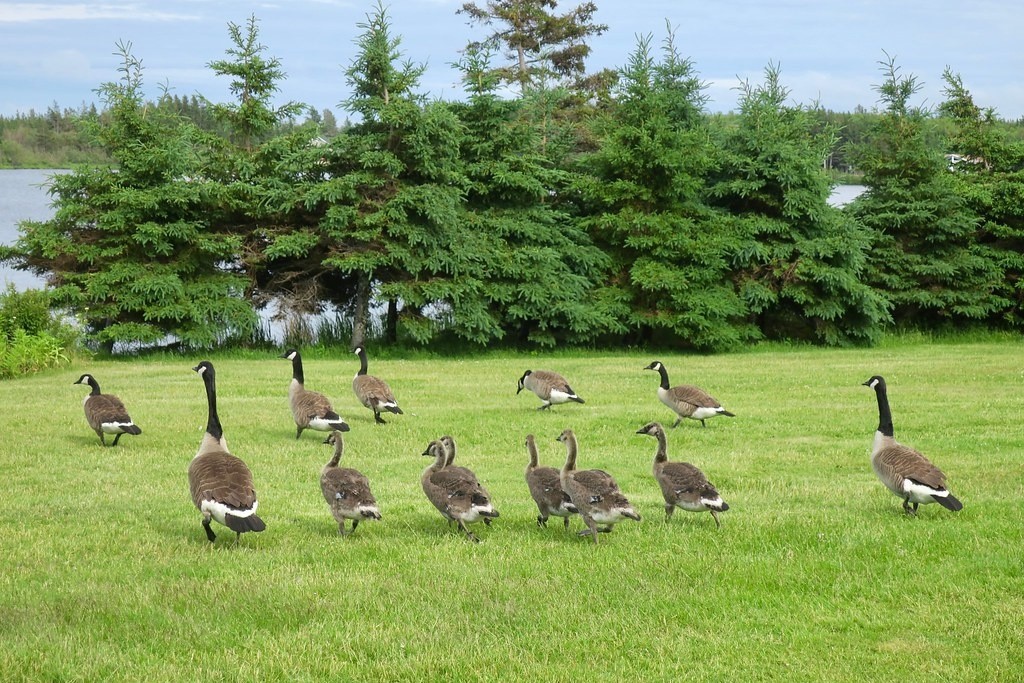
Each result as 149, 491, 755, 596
187, 361, 266, 547
72, 374, 141, 448
420, 436, 500, 543
643, 361, 736, 429
516, 370, 586, 412
523, 434, 581, 532
861, 375, 963, 518
319, 432, 382, 536
555, 428, 643, 546
347, 346, 403, 426
277, 349, 350, 439
635, 422, 730, 529
